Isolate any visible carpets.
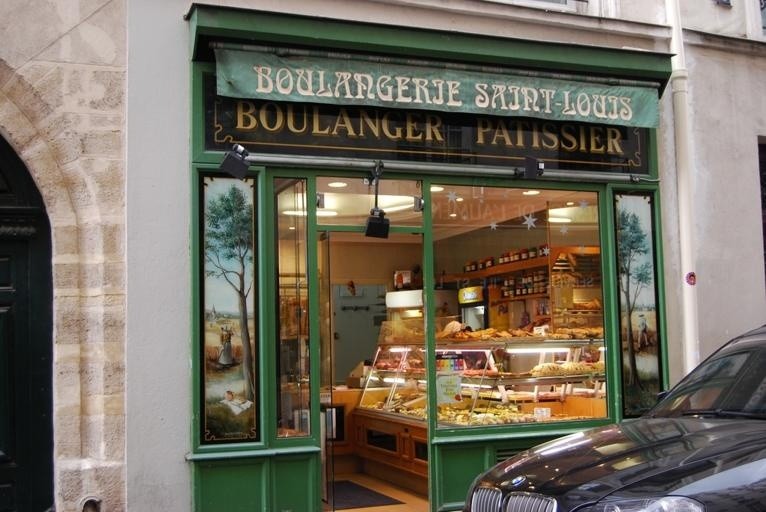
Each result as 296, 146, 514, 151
322, 479, 403, 508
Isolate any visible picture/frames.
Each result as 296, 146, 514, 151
198, 167, 261, 444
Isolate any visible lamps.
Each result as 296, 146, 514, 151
365, 160, 390, 238
515, 155, 544, 181
220, 142, 250, 179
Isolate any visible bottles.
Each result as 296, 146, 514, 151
509, 288, 514, 298
533, 282, 539, 294
521, 274, 527, 283
527, 283, 533, 294
533, 271, 538, 281
504, 253, 510, 263
528, 248, 537, 258
396, 273, 403, 291
470, 262, 477, 270
466, 263, 471, 271
527, 274, 532, 282
504, 278, 509, 287
514, 251, 520, 260
510, 251, 514, 261
515, 283, 521, 296
521, 283, 527, 295
543, 244, 548, 255
501, 287, 508, 297
538, 271, 544, 281
498, 254, 504, 263
539, 282, 546, 293
480, 260, 485, 269
509, 278, 515, 286
539, 245, 543, 256
485, 256, 495, 268
521, 249, 528, 259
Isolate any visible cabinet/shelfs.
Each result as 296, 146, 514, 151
279, 273, 323, 387
435, 256, 551, 329
351, 340, 608, 499
282, 387, 403, 473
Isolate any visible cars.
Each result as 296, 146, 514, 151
463, 324, 765, 512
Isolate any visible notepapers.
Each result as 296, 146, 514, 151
436, 375, 464, 405
